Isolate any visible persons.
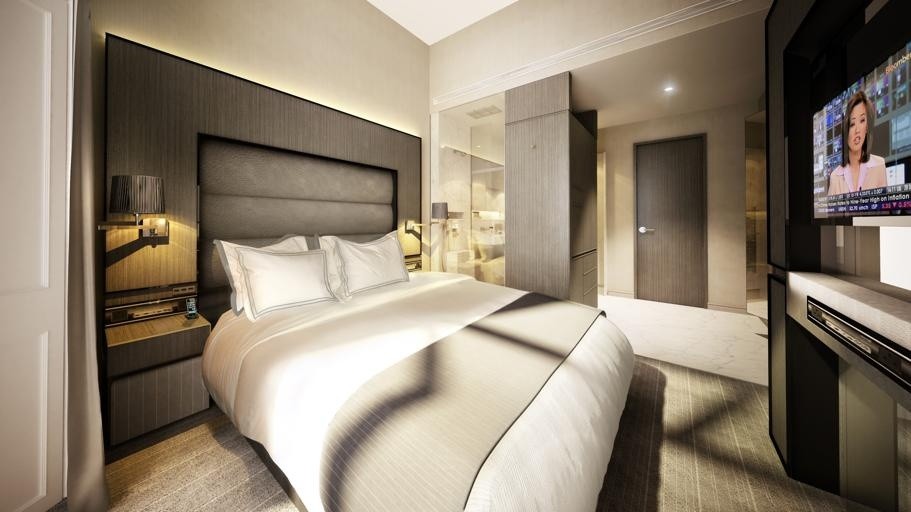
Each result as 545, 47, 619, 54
827, 93, 887, 195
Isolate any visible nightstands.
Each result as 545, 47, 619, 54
105, 311, 211, 446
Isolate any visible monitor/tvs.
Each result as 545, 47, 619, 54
807, 41, 911, 226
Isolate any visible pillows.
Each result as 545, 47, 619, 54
212, 232, 309, 316
327, 236, 409, 296
234, 246, 343, 322
318, 238, 351, 302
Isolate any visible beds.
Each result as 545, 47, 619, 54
200, 270, 634, 512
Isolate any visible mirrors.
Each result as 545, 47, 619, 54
744, 110, 769, 319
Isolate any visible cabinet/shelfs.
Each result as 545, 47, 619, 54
571, 251, 597, 307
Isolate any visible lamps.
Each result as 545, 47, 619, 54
405, 203, 448, 232
98, 175, 168, 239
